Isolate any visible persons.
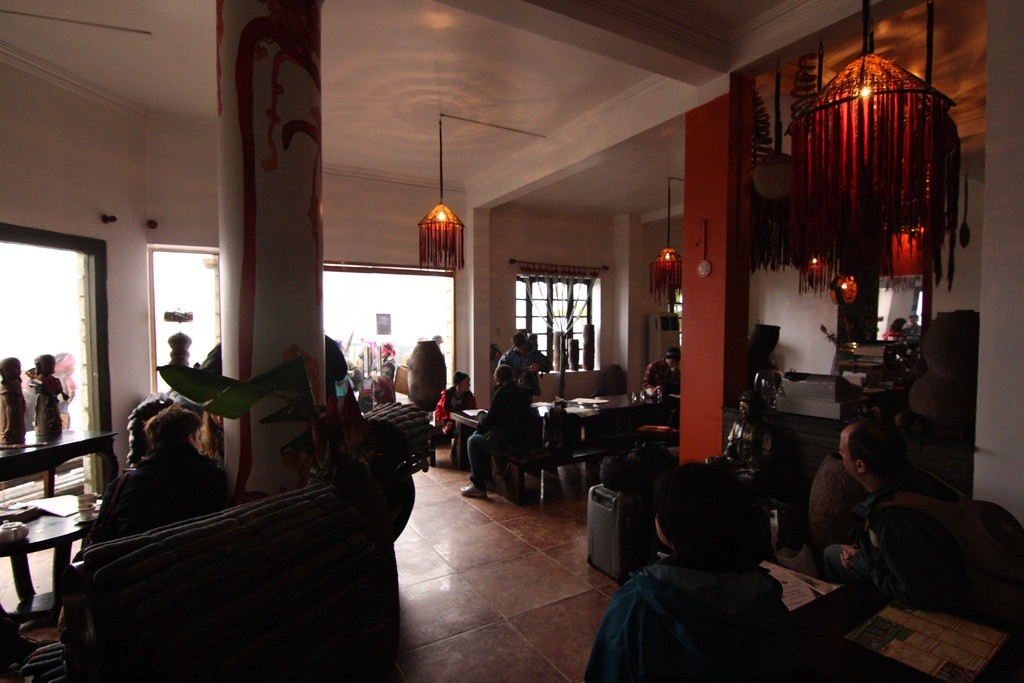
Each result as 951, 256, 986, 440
323, 331, 444, 412
822, 413, 1001, 627
0, 355, 62, 449
582, 460, 801, 683
436, 370, 479, 466
723, 390, 776, 468
55, 404, 229, 628
497, 333, 553, 403
865, 345, 927, 428
642, 347, 681, 398
459, 365, 531, 499
884, 310, 925, 341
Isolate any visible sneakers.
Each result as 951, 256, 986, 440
467, 480, 495, 490
460, 482, 487, 498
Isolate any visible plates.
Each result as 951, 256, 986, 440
74, 514, 99, 523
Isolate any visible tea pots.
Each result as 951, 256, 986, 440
0, 520, 29, 545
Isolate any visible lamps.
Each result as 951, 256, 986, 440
417, 114, 465, 273
650, 177, 682, 304
788, 0, 962, 302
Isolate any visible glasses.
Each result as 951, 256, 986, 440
514, 342, 524, 347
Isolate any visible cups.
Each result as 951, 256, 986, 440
79, 493, 97, 504
79, 504, 95, 520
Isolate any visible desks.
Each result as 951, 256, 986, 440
0, 431, 119, 498
0, 496, 102, 624
448, 394, 1024, 683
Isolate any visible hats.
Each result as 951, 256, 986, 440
666, 347, 681, 360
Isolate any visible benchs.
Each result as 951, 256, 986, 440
20, 481, 378, 683
496, 431, 640, 502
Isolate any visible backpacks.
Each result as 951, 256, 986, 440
872, 465, 1024, 632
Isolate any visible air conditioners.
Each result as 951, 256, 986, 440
649, 312, 679, 362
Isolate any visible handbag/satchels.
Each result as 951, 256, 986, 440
87, 471, 132, 545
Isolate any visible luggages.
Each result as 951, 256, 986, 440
588, 482, 652, 586
